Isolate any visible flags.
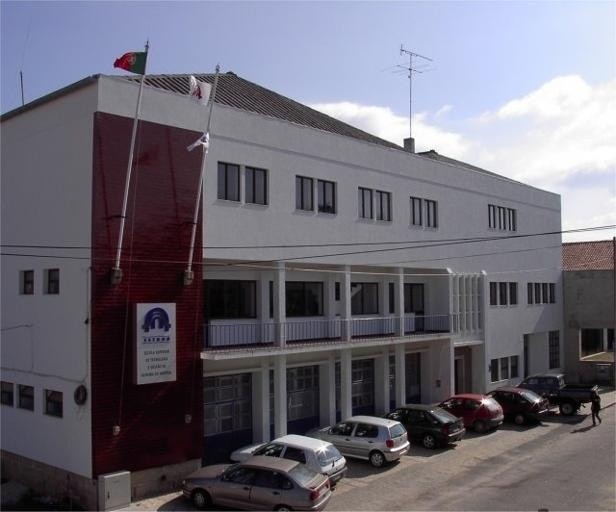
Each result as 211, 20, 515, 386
189, 74, 212, 106
113, 52, 146, 75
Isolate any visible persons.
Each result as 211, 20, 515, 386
591, 391, 601, 426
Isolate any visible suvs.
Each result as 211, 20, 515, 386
308, 414, 411, 467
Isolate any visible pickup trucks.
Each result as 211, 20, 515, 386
519, 373, 599, 418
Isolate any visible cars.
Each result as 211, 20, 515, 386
487, 386, 550, 429
230, 434, 348, 485
180, 454, 332, 511
438, 392, 505, 433
381, 403, 466, 448
